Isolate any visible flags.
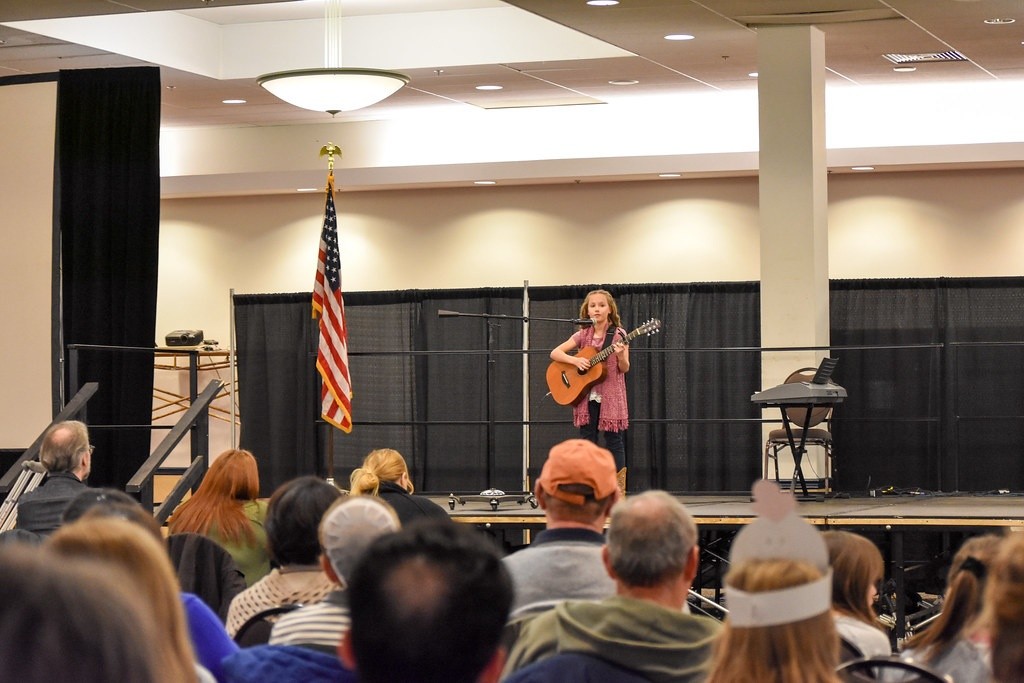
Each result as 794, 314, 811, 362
312, 173, 353, 433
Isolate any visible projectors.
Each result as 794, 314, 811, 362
166, 330, 204, 346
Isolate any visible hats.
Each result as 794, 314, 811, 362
539, 438, 618, 505
324, 499, 396, 584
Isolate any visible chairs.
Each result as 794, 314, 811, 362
764, 367, 833, 497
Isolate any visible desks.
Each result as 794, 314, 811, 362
150, 349, 240, 426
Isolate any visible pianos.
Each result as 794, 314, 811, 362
750, 381, 848, 503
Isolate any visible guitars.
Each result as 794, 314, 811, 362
546, 317, 662, 407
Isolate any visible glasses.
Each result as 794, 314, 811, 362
85, 445, 93, 456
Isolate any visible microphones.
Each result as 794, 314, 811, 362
575, 318, 597, 325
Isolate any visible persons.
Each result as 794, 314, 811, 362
341, 521, 629, 682
550, 289, 629, 501
0, 476, 354, 683
886, 536, 1003, 683
168, 449, 273, 587
703, 557, 845, 683
969, 532, 1024, 683
270, 493, 401, 646
349, 449, 453, 526
16, 421, 95, 534
496, 439, 690, 623
821, 530, 892, 674
497, 490, 724, 683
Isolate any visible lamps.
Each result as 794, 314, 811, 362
256, 68, 411, 116
